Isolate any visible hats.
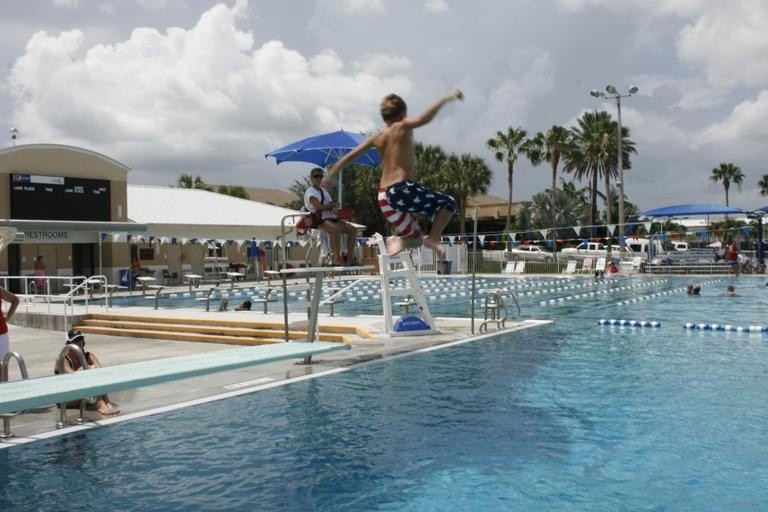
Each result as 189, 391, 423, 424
67, 328, 85, 342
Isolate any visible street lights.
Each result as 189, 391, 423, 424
590, 85, 638, 253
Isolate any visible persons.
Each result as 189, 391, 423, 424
322, 87, 466, 266
326, 249, 362, 279
687, 285, 693, 294
725, 240, 740, 275
249, 237, 261, 282
608, 262, 618, 272
0, 284, 20, 381
54, 328, 121, 416
33, 255, 47, 295
234, 300, 251, 310
741, 260, 749, 273
302, 167, 359, 268
211, 281, 223, 299
724, 284, 736, 296
130, 256, 141, 290
217, 299, 229, 311
694, 286, 700, 294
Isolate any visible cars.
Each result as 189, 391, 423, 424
604, 236, 728, 259
505, 242, 555, 265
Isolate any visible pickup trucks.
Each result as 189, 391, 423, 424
562, 239, 611, 261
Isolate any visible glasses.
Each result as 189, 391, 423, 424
312, 173, 324, 179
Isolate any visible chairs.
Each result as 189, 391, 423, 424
560, 257, 605, 276
501, 260, 525, 274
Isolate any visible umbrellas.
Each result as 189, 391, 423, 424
754, 259, 766, 274
259, 126, 387, 213
